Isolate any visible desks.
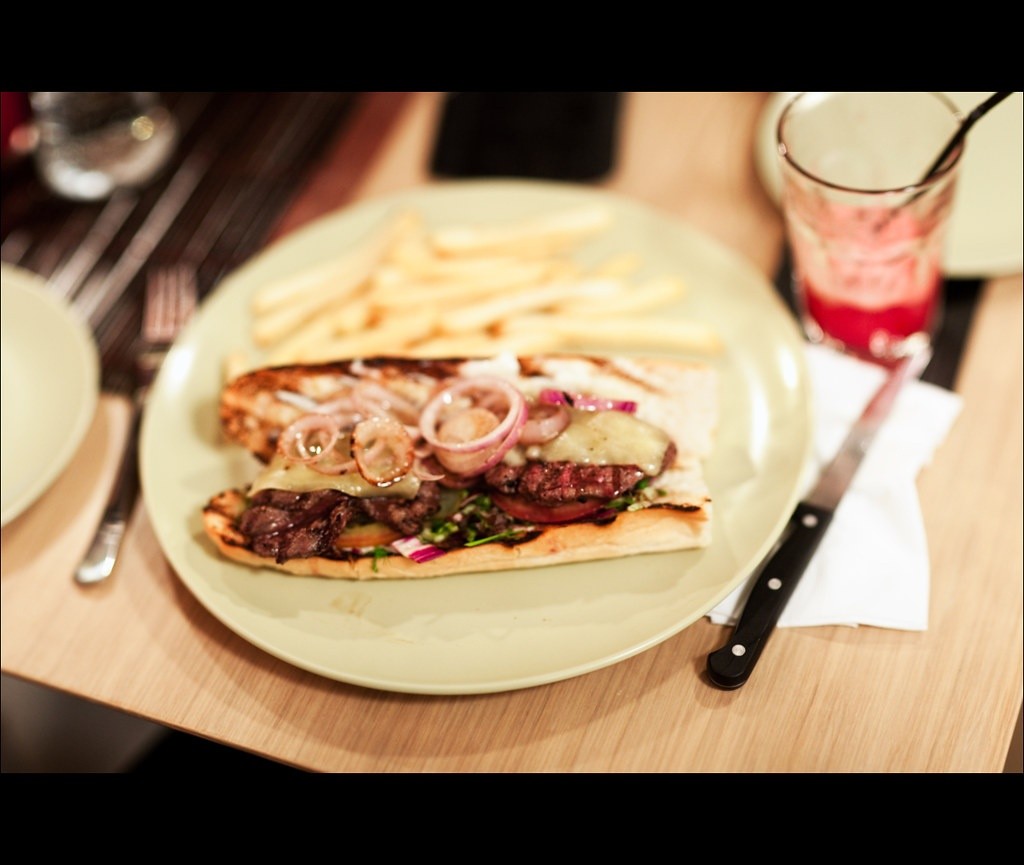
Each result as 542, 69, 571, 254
0, 92, 1024, 773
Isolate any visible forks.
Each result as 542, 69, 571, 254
72, 262, 200, 585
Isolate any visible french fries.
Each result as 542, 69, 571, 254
220, 210, 725, 385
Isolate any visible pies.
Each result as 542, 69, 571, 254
201, 350, 709, 579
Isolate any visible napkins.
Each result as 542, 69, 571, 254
702, 363, 965, 629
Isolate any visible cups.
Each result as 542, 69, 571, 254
774, 93, 966, 377
26, 91, 179, 201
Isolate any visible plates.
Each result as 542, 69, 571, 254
0, 260, 102, 531
139, 179, 821, 696
747, 91, 1023, 282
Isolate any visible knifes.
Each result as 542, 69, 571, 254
706, 352, 922, 690
